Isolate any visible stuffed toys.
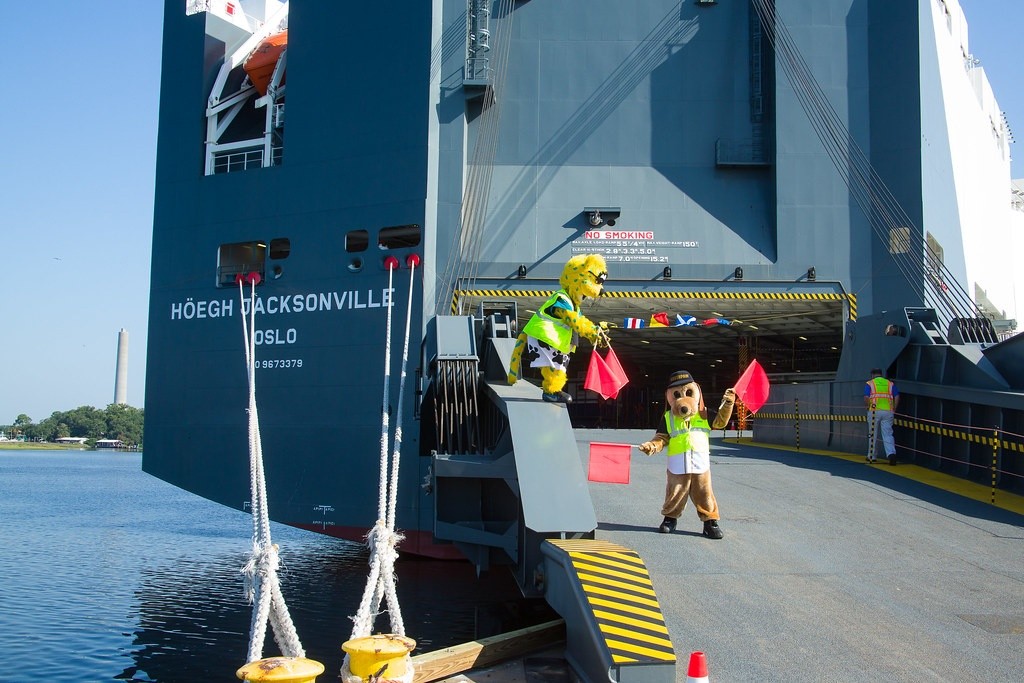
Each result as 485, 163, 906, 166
509, 254, 612, 403
639, 371, 735, 539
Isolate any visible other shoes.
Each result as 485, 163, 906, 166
889, 454, 896, 466
866, 458, 876, 462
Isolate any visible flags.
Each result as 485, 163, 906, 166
734, 358, 770, 414
588, 442, 631, 484
624, 318, 644, 329
703, 318, 730, 327
584, 350, 620, 399
649, 313, 669, 327
676, 314, 697, 327
605, 350, 629, 399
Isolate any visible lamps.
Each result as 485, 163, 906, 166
809, 265, 817, 280
885, 324, 900, 336
735, 266, 743, 278
518, 264, 526, 277
663, 264, 672, 277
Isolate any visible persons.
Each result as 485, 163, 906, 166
864, 367, 899, 465
